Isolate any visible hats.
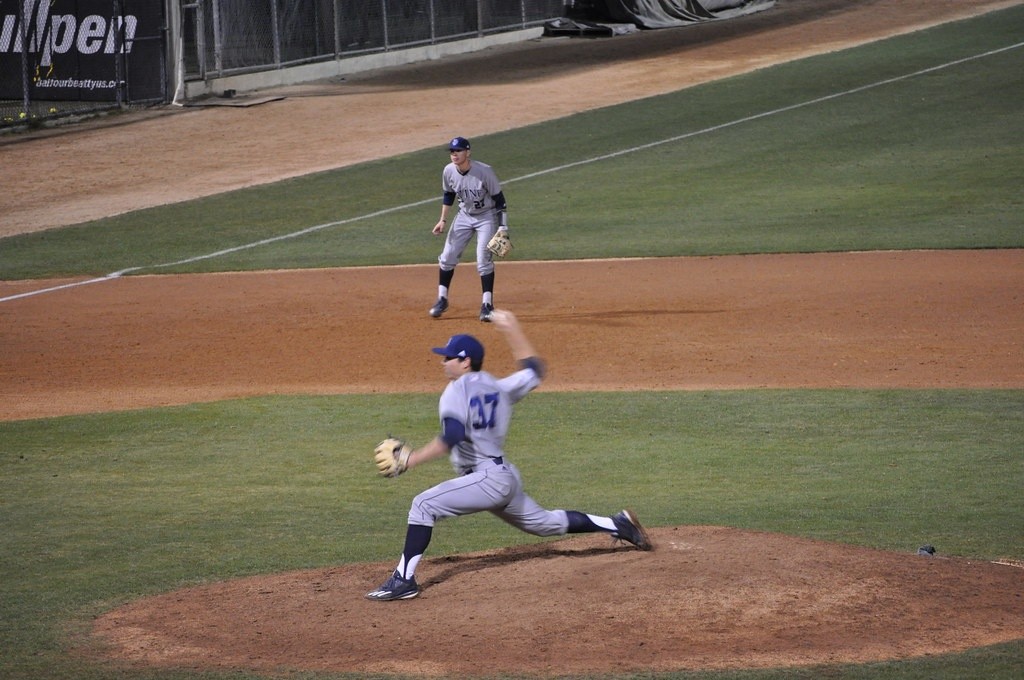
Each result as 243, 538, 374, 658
448, 137, 470, 150
431, 334, 482, 363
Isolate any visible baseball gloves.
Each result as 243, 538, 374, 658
373, 432, 414, 479
486, 225, 515, 264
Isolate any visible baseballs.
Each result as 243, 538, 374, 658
492, 312, 505, 322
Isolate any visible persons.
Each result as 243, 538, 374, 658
363, 310, 653, 603
427, 137, 511, 321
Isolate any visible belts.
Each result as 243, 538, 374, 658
465, 457, 502, 475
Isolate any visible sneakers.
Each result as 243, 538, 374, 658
429, 296, 448, 317
480, 303, 494, 322
609, 509, 651, 551
364, 569, 418, 601
